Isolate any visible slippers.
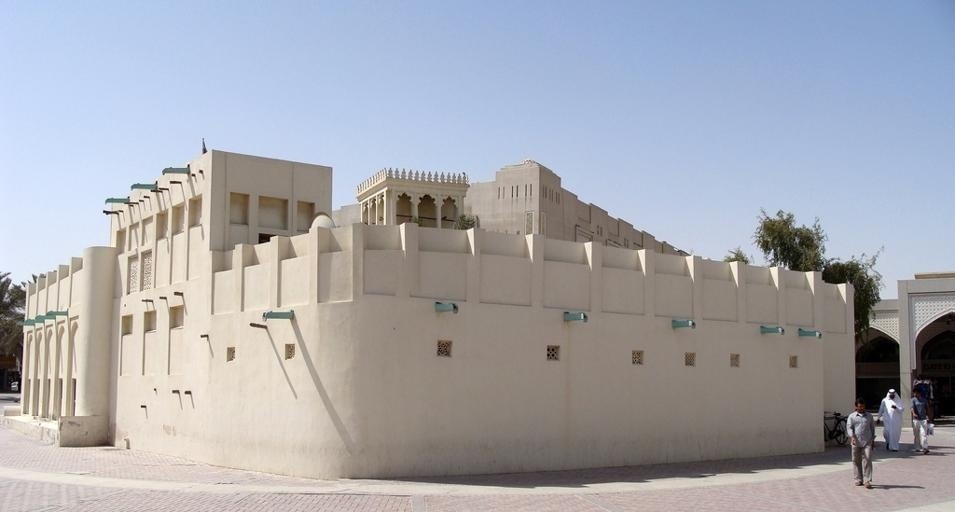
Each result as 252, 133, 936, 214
924, 450, 929, 454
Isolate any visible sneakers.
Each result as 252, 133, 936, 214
854, 480, 863, 486
864, 481, 871, 488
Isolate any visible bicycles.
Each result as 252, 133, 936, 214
823, 409, 850, 448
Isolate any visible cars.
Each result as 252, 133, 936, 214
10, 381, 19, 392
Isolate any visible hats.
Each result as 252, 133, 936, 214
889, 388, 895, 393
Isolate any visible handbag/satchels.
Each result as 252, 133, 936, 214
926, 422, 935, 436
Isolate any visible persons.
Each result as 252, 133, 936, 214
846, 397, 876, 489
876, 389, 904, 452
909, 374, 935, 455
